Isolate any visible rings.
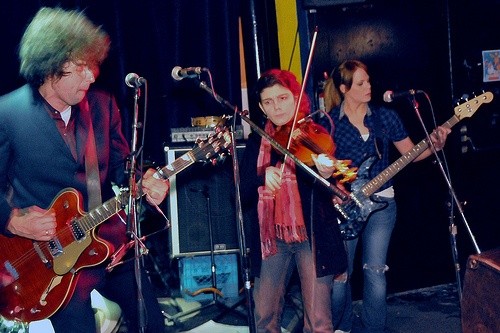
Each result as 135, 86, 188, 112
45, 230, 49, 237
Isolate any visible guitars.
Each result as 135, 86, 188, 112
333, 89, 493, 241
0, 126, 233, 323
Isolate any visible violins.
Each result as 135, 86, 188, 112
274, 116, 358, 184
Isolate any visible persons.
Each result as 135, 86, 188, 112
0, 7, 171, 333
316, 58, 451, 333
239, 68, 349, 333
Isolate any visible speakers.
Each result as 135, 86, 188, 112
461, 248, 500, 333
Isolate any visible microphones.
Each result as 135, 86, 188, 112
383, 89, 416, 102
125, 73, 144, 87
171, 65, 208, 80
